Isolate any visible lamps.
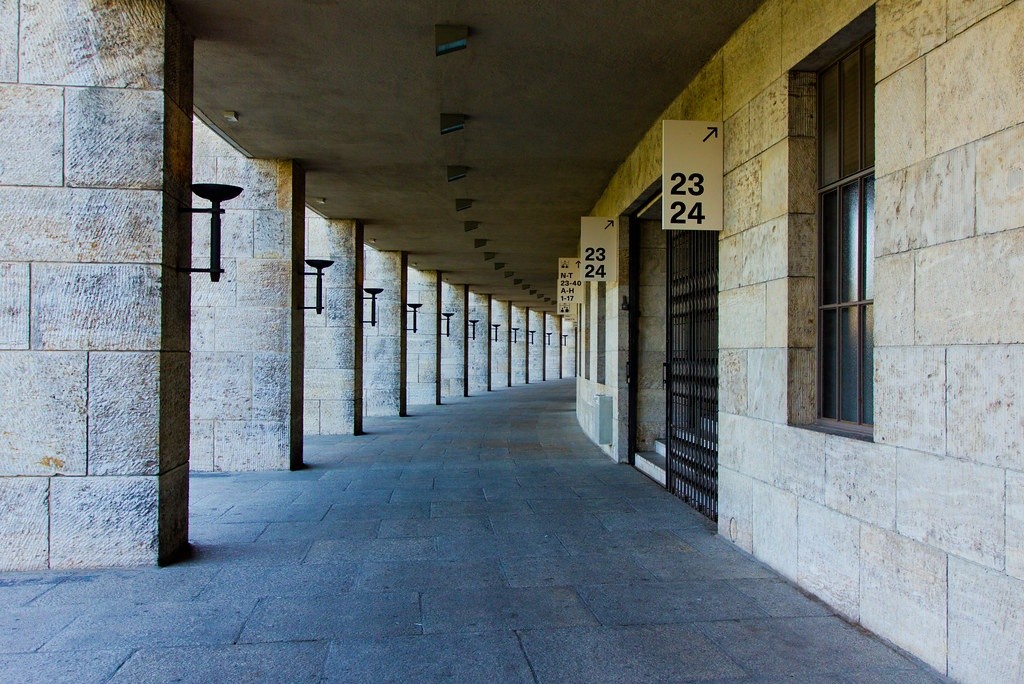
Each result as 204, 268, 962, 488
490, 325, 500, 342
561, 335, 568, 346
495, 263, 505, 270
440, 313, 454, 337
435, 24, 468, 55
447, 165, 466, 182
464, 221, 479, 231
504, 271, 557, 305
528, 331, 536, 344
511, 328, 520, 343
484, 252, 495, 261
474, 239, 487, 248
405, 304, 422, 333
467, 320, 479, 340
178, 184, 243, 282
360, 289, 383, 326
545, 333, 552, 345
456, 199, 472, 211
298, 260, 334, 314
440, 113, 465, 135
